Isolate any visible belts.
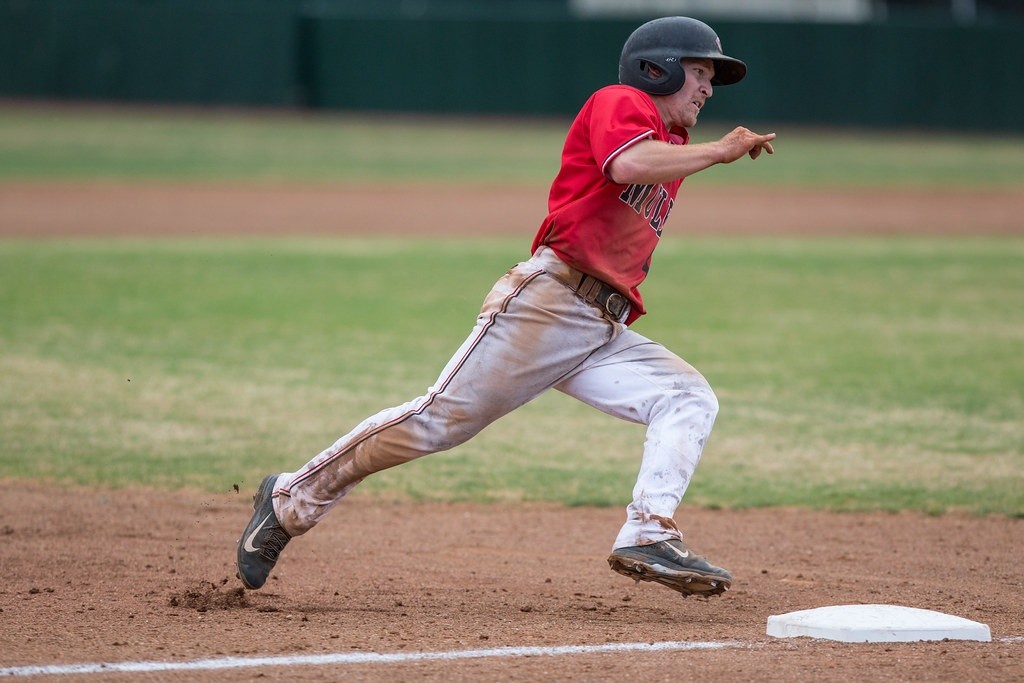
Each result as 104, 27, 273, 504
577, 274, 630, 321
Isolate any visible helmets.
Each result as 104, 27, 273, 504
618, 15, 747, 96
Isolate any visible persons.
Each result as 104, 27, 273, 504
237, 15, 777, 602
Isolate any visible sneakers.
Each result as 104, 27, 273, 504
608, 537, 732, 601
237, 476, 291, 590
